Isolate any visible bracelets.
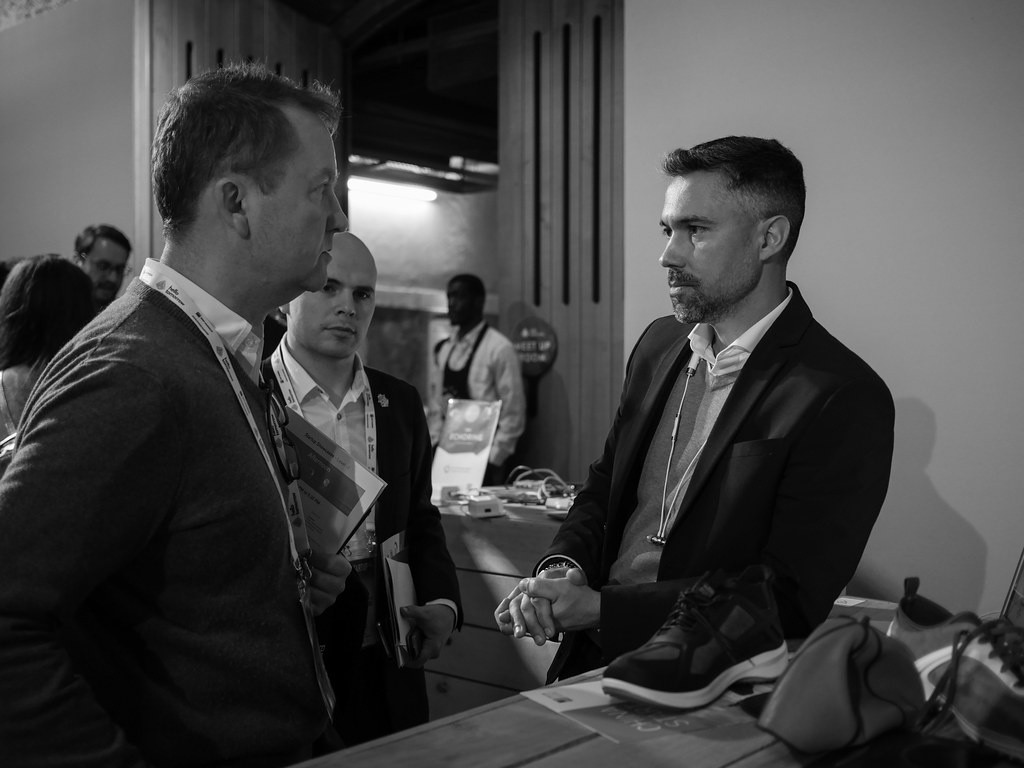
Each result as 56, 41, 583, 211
539, 561, 579, 575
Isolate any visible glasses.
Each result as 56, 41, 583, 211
265, 389, 301, 485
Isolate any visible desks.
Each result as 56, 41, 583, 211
289, 597, 899, 768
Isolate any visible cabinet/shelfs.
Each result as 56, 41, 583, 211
421, 500, 567, 723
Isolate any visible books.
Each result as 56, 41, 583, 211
283, 404, 388, 556
383, 557, 420, 668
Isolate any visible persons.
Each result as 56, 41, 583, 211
494, 135, 895, 686
258, 231, 464, 747
0, 69, 347, 768
426, 273, 526, 487
0, 255, 92, 471
72, 223, 132, 313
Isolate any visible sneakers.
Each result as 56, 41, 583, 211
600, 563, 787, 710
886, 577, 1024, 759
